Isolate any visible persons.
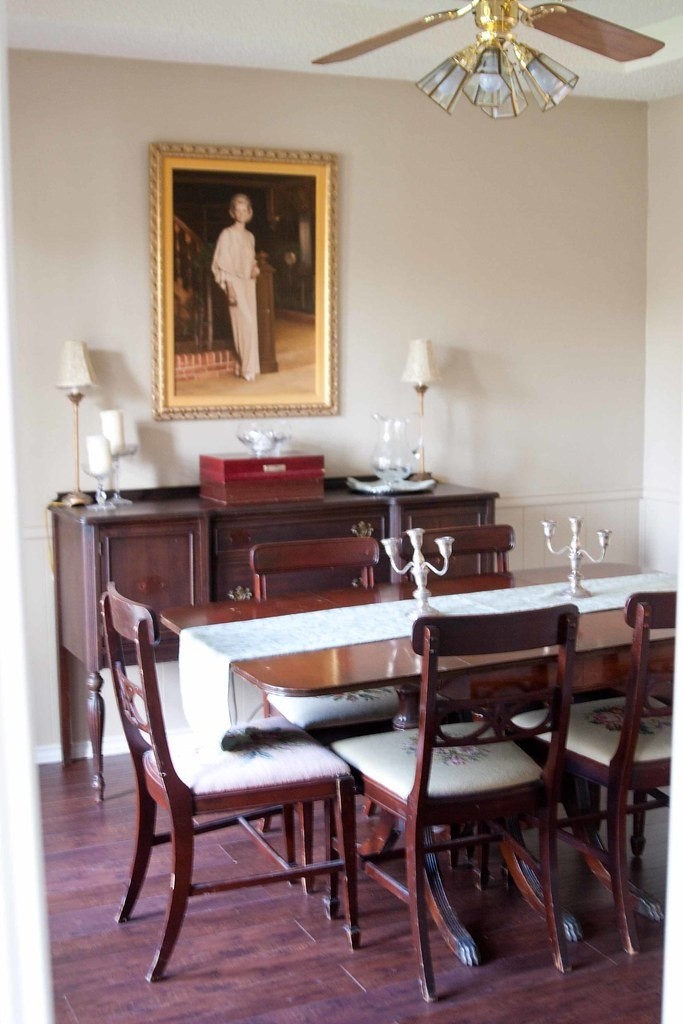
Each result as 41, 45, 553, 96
211, 194, 261, 382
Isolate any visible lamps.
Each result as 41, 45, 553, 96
415, 32, 579, 119
402, 338, 439, 482
54, 340, 99, 505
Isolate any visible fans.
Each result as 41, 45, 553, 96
312, 0, 665, 64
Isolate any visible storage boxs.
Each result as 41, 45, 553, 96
200, 451, 326, 504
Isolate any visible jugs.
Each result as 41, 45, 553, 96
371, 412, 421, 486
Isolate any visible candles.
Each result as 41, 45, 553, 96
86, 435, 111, 475
100, 410, 124, 453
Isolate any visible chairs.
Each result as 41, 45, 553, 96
100, 524, 680, 1003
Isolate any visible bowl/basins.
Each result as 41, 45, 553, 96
237, 423, 293, 457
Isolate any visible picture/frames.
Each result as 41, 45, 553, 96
149, 140, 339, 421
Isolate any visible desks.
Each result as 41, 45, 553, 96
159, 562, 679, 967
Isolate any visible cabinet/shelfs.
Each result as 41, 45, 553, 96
52, 475, 500, 804
